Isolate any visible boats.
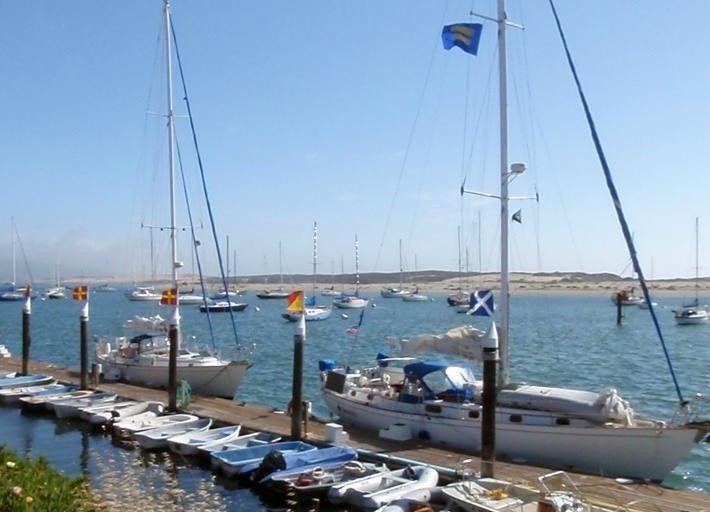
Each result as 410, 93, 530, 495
199, 301, 249, 313
39, 264, 214, 305
670, 308, 709, 325
320, 286, 341, 296
254, 460, 625, 512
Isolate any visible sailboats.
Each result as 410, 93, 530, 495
220, 248, 249, 295
399, 254, 429, 303
256, 255, 296, 299
318, 0, 710, 482
93, 3, 257, 400
635, 263, 658, 310
281, 219, 333, 321
680, 216, 701, 309
332, 234, 370, 309
209, 236, 237, 299
446, 210, 483, 307
608, 256, 650, 305
380, 238, 419, 299
2, 217, 38, 301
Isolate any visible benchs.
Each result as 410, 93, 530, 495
441, 22, 483, 57
160, 288, 177, 304
23, 284, 31, 295
72, 286, 88, 300
466, 289, 493, 317
286, 290, 304, 312
